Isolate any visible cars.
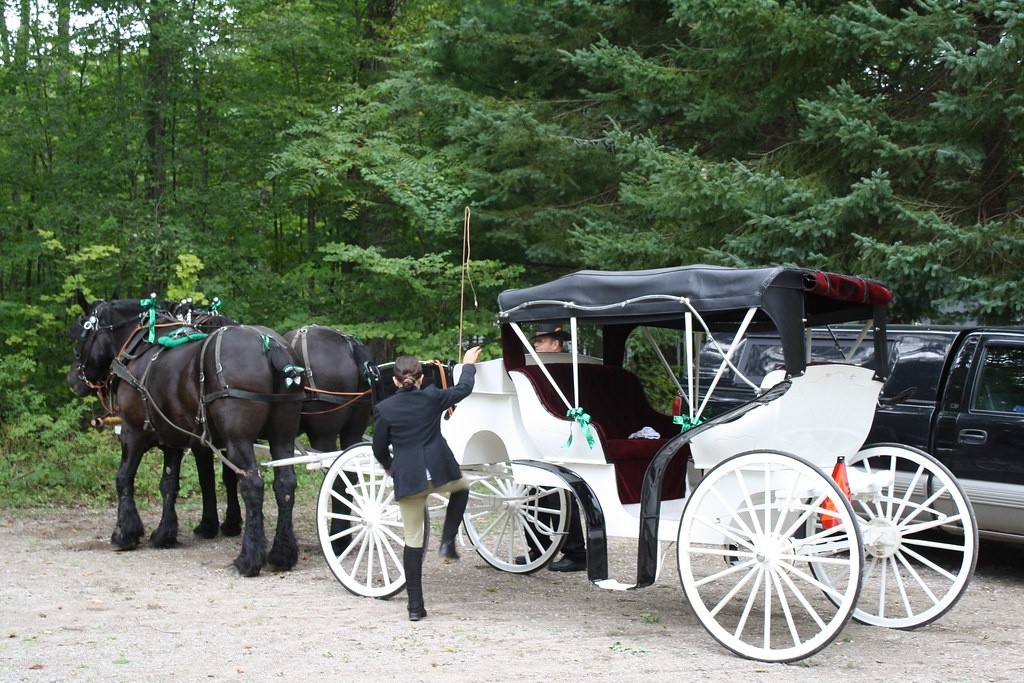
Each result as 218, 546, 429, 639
673, 325, 1024, 542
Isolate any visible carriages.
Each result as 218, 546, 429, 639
67, 265, 979, 664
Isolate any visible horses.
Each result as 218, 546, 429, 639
170, 299, 385, 556
66, 298, 305, 577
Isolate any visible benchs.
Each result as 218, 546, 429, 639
508, 365, 687, 505
691, 362, 884, 475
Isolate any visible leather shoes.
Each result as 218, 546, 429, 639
515, 550, 539, 565
547, 555, 587, 572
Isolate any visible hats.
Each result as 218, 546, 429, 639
527, 320, 571, 342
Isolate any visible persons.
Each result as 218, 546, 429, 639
515, 322, 588, 572
372, 346, 481, 621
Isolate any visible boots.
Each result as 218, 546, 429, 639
438, 488, 469, 561
403, 545, 427, 621
